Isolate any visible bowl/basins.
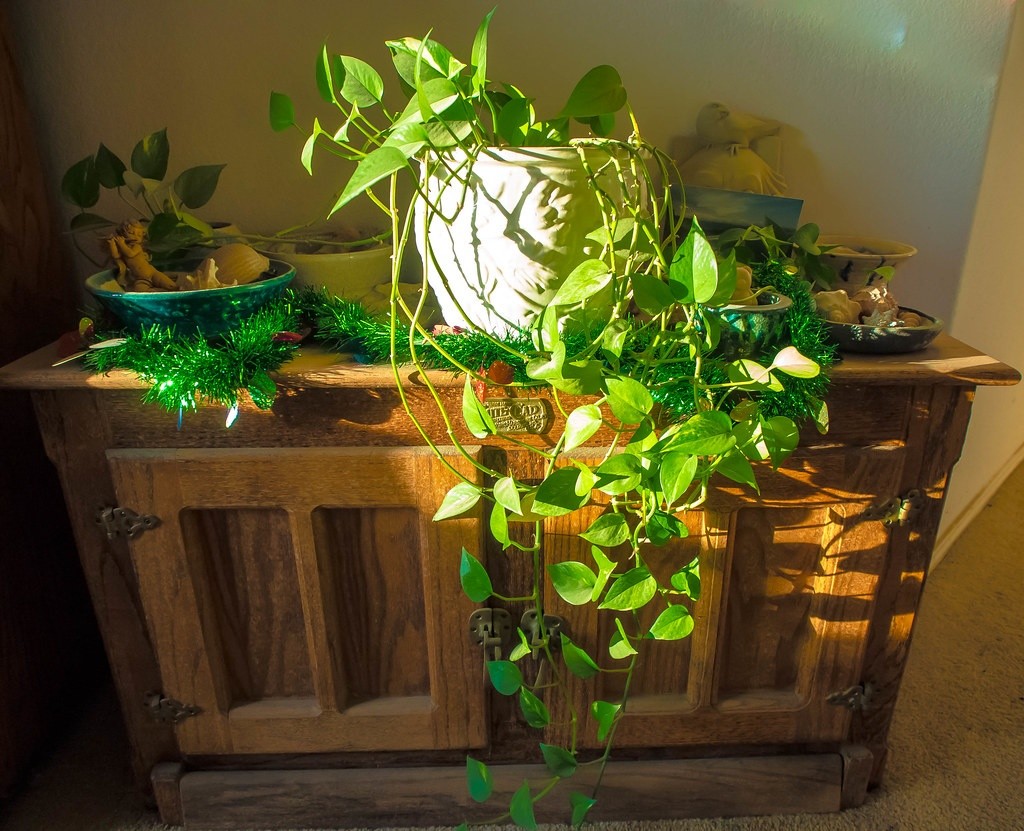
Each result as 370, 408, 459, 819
794, 235, 917, 298
254, 231, 394, 303
86, 260, 298, 339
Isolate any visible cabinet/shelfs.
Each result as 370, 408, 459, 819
0, 328, 1022, 831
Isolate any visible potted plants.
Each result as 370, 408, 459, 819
60, 7, 829, 831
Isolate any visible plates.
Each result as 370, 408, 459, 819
819, 306, 943, 353
